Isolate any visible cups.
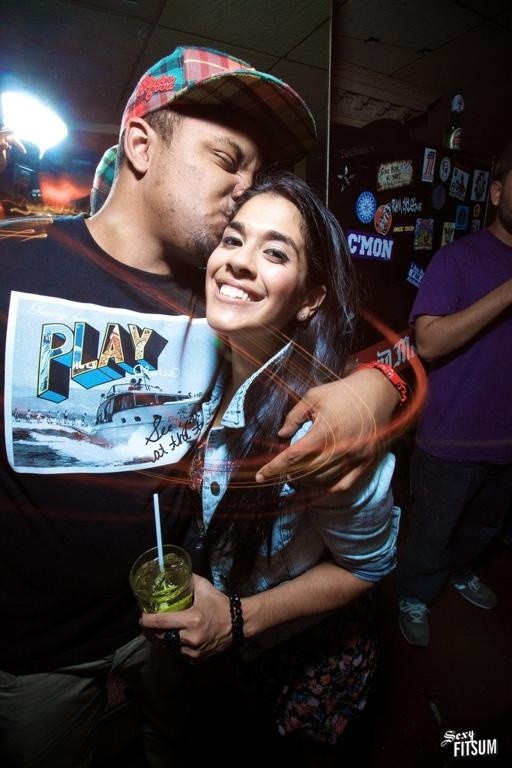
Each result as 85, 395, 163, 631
128, 545, 194, 614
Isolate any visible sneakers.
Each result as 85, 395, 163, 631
447, 571, 497, 612
395, 592, 432, 650
139, 714, 177, 768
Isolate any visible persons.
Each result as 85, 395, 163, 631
0, 44, 511, 768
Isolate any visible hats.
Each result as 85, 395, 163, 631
118, 43, 319, 168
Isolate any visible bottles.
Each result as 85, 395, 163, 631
443, 92, 465, 151
